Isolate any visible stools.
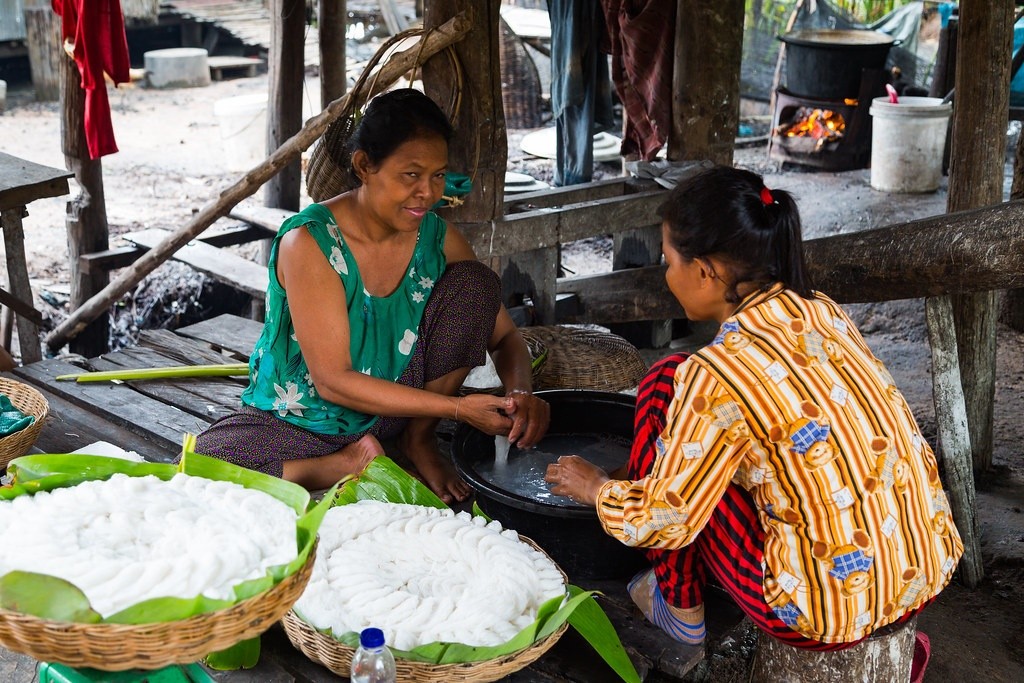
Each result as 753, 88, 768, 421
749, 612, 918, 683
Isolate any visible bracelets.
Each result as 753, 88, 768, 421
454, 395, 463, 423
505, 388, 534, 401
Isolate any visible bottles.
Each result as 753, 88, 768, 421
349, 628, 397, 682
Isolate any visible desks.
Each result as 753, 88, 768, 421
0, 148, 75, 367
499, 4, 552, 57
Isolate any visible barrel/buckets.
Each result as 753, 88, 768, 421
868, 95, 951, 192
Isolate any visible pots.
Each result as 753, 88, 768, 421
451, 390, 639, 579
776, 28, 904, 101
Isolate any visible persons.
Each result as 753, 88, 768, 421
543, 164, 965, 653
191, 87, 551, 509
